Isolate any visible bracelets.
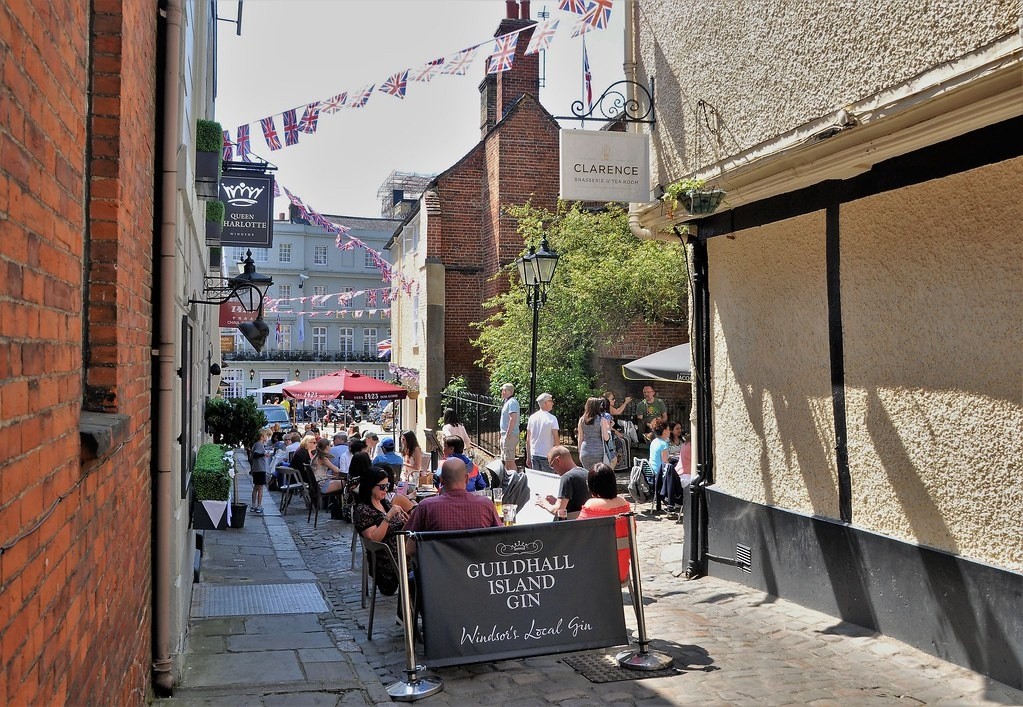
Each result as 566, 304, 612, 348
384, 517, 391, 523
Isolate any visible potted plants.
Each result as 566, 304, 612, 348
196, 119, 225, 272
205, 395, 269, 529
660, 177, 732, 220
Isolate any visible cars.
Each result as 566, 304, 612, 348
317, 409, 353, 423
257, 405, 293, 431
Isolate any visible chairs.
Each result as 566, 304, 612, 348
634, 458, 683, 523
275, 452, 637, 653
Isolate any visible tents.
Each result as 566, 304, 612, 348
251, 379, 303, 409
283, 366, 407, 436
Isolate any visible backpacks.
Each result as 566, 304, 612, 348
628, 457, 655, 504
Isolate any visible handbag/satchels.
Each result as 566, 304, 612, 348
603, 450, 618, 472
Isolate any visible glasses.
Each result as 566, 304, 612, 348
608, 397, 615, 401
550, 456, 560, 469
377, 482, 390, 490
285, 438, 291, 441
310, 442, 318, 444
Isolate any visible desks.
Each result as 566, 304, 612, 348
416, 492, 436, 503
339, 470, 349, 479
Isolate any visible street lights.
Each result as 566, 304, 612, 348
516, 231, 562, 475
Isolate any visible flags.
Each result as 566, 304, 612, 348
585, 44, 593, 117
298, 315, 304, 343
223, 31, 522, 163
524, 0, 620, 58
378, 340, 393, 358
275, 315, 280, 343
219, 156, 423, 320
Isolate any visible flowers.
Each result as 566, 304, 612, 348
388, 362, 420, 394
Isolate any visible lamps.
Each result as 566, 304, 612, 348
295, 369, 300, 378
203, 248, 274, 312
250, 369, 255, 383
183, 283, 269, 354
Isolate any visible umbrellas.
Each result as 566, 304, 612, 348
621, 342, 691, 383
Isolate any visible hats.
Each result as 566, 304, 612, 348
360, 431, 377, 441
379, 437, 395, 448
331, 431, 348, 437
499, 383, 515, 391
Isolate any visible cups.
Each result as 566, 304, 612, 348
477, 488, 513, 526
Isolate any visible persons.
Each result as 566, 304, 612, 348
353, 463, 419, 628
535, 445, 593, 523
249, 395, 486, 518
577, 385, 691, 512
499, 382, 519, 472
526, 393, 560, 474
575, 462, 637, 584
401, 457, 503, 556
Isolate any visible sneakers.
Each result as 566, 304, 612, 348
250, 506, 257, 512
256, 507, 264, 513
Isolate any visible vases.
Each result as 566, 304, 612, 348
407, 392, 418, 399
